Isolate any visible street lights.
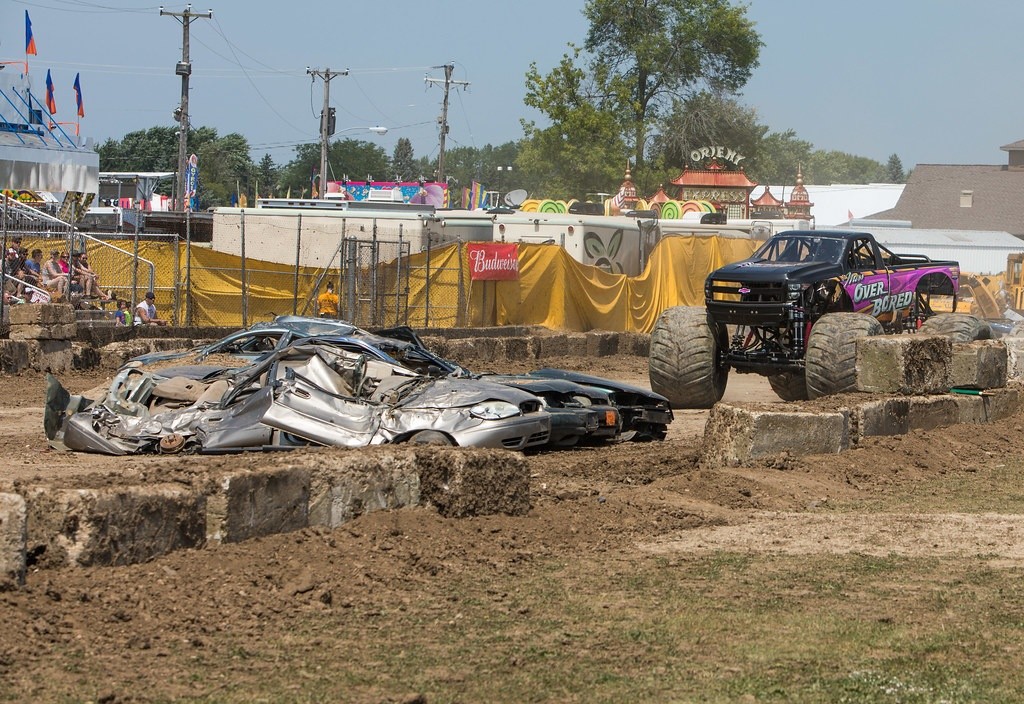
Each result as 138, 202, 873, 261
497, 167, 503, 191
323, 124, 388, 198
507, 166, 512, 192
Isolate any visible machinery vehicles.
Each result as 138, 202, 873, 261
922, 251, 1024, 323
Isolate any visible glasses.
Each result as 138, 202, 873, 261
82, 257, 87, 260
22, 253, 28, 255
7, 297, 12, 299
62, 256, 69, 258
149, 297, 155, 299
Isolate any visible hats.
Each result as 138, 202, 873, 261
50, 249, 59, 254
7, 248, 16, 254
73, 250, 79, 255
146, 292, 154, 297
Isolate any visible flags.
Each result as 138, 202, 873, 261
73, 73, 84, 116
26, 9, 37, 55
46, 69, 56, 114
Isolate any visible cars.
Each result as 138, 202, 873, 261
649, 230, 960, 410
42, 314, 673, 457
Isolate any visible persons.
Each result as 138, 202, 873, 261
0, 237, 43, 324
43, 249, 109, 300
133, 292, 167, 325
316, 283, 338, 319
116, 300, 136, 326
